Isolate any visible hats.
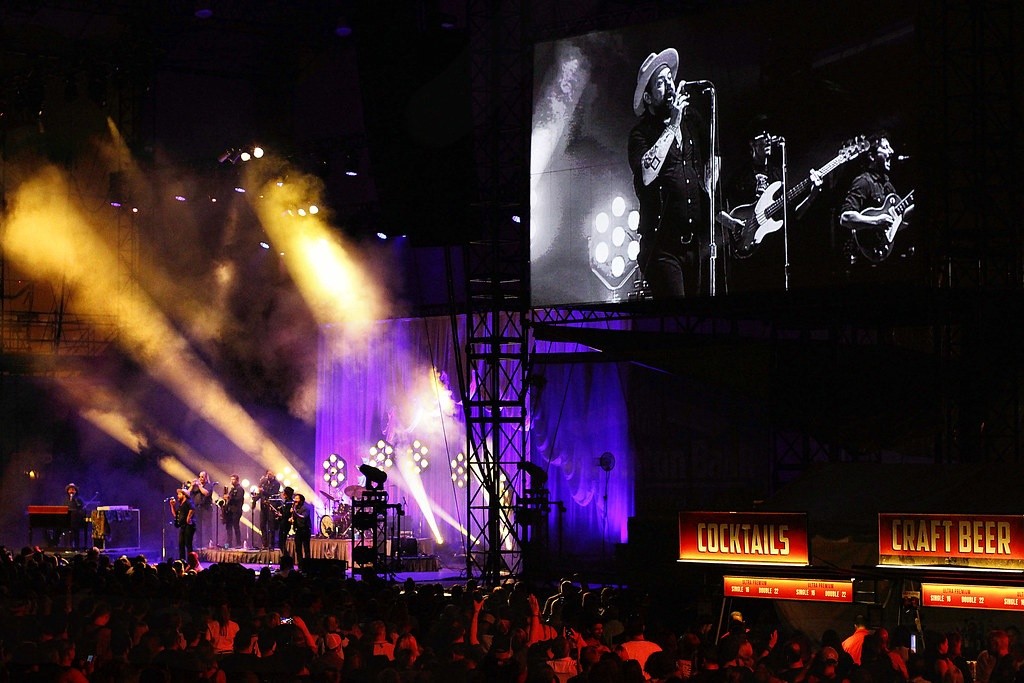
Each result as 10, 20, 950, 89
326, 633, 340, 650
177, 489, 190, 499
65, 483, 78, 497
819, 647, 838, 665
633, 47, 680, 118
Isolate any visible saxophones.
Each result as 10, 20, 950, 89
216, 485, 229, 524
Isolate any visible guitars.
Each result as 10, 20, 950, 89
723, 134, 871, 260
851, 189, 915, 261
262, 499, 279, 513
290, 500, 298, 531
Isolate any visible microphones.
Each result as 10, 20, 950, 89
403, 497, 408, 507
70, 494, 73, 501
213, 482, 218, 484
773, 135, 785, 146
679, 79, 710, 94
166, 497, 174, 500
898, 156, 910, 161
325, 530, 330, 533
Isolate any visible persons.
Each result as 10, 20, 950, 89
627, 48, 823, 299
0, 471, 1024, 683
838, 130, 915, 272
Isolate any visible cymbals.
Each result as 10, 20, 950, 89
319, 490, 335, 500
343, 484, 366, 499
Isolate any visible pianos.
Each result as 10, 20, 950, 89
26, 505, 92, 552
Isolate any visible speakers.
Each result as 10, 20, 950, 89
390, 537, 418, 558
297, 557, 347, 581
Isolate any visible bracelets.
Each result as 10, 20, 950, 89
532, 614, 538, 617
667, 120, 678, 133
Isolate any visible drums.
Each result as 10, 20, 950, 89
337, 503, 349, 514
344, 512, 352, 524
320, 514, 343, 538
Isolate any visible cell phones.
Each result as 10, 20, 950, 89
87, 655, 94, 663
280, 618, 294, 625
